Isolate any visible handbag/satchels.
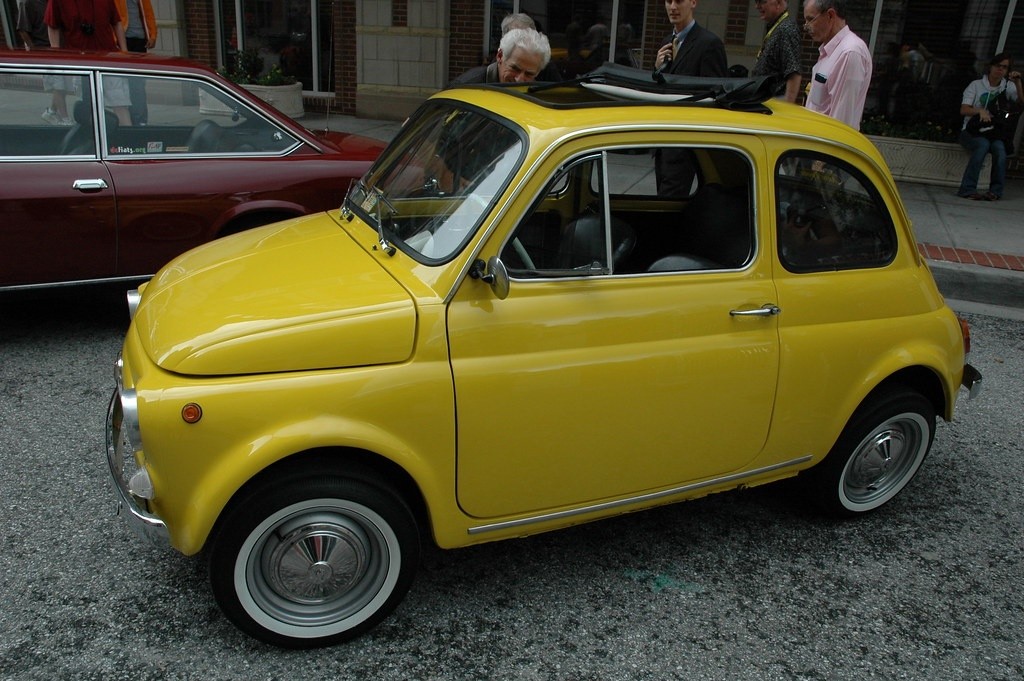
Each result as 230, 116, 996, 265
966, 93, 1024, 145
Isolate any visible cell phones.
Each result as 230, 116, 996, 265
815, 73, 828, 83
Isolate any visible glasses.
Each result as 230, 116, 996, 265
994, 63, 1011, 69
803, 13, 823, 31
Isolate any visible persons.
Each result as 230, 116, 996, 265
567, 14, 641, 70
871, 39, 924, 115
652, 0, 728, 198
794, 0, 873, 188
752, 0, 802, 175
781, 188, 842, 266
492, 13, 562, 81
934, 50, 978, 129
17, 0, 158, 126
401, 28, 551, 197
955, 54, 1024, 200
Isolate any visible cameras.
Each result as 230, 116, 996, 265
82, 23, 95, 35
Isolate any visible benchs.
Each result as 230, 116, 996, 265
182, 119, 259, 152
689, 184, 883, 269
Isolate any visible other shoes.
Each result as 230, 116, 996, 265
966, 192, 1000, 200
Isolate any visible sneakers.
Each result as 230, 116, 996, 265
42, 106, 75, 125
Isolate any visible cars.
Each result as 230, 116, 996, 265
105, 83, 978, 656
1, 46, 468, 306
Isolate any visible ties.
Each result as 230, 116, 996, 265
671, 37, 678, 61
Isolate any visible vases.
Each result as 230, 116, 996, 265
238, 82, 306, 118
863, 134, 991, 190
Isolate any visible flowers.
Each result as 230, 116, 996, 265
860, 115, 962, 145
215, 47, 298, 87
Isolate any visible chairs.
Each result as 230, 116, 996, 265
646, 252, 728, 271
565, 218, 636, 265
58, 100, 118, 155
